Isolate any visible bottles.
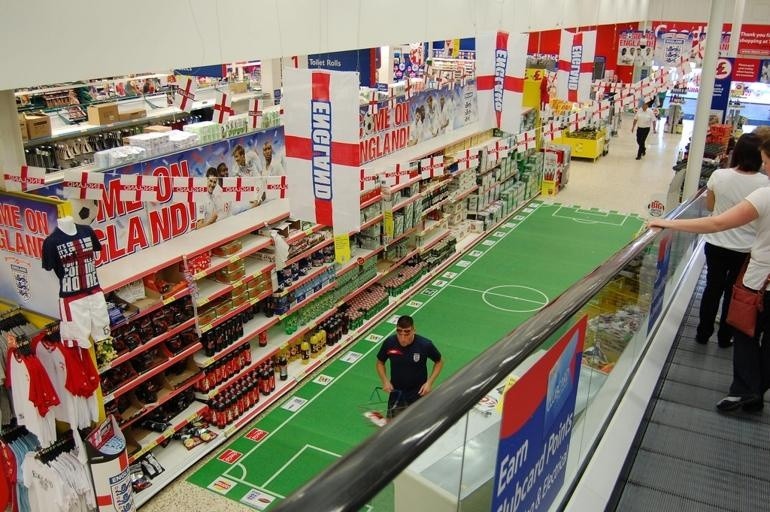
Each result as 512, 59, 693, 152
193, 295, 351, 431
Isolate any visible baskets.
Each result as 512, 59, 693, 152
358, 386, 408, 426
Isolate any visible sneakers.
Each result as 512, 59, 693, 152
695, 332, 709, 344
716, 391, 764, 415
718, 338, 734, 348
636, 149, 645, 160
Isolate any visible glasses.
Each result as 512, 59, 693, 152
396, 328, 412, 333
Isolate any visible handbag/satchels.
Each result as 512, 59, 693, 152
725, 285, 763, 336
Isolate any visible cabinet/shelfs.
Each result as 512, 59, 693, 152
0, 74, 543, 512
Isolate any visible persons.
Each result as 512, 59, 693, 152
647, 139, 769, 413
197, 143, 285, 229
377, 316, 443, 423
621, 48, 653, 83
408, 90, 460, 146
42, 215, 111, 350
695, 132, 769, 347
630, 101, 656, 160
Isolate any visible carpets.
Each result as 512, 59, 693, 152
185, 197, 649, 512
